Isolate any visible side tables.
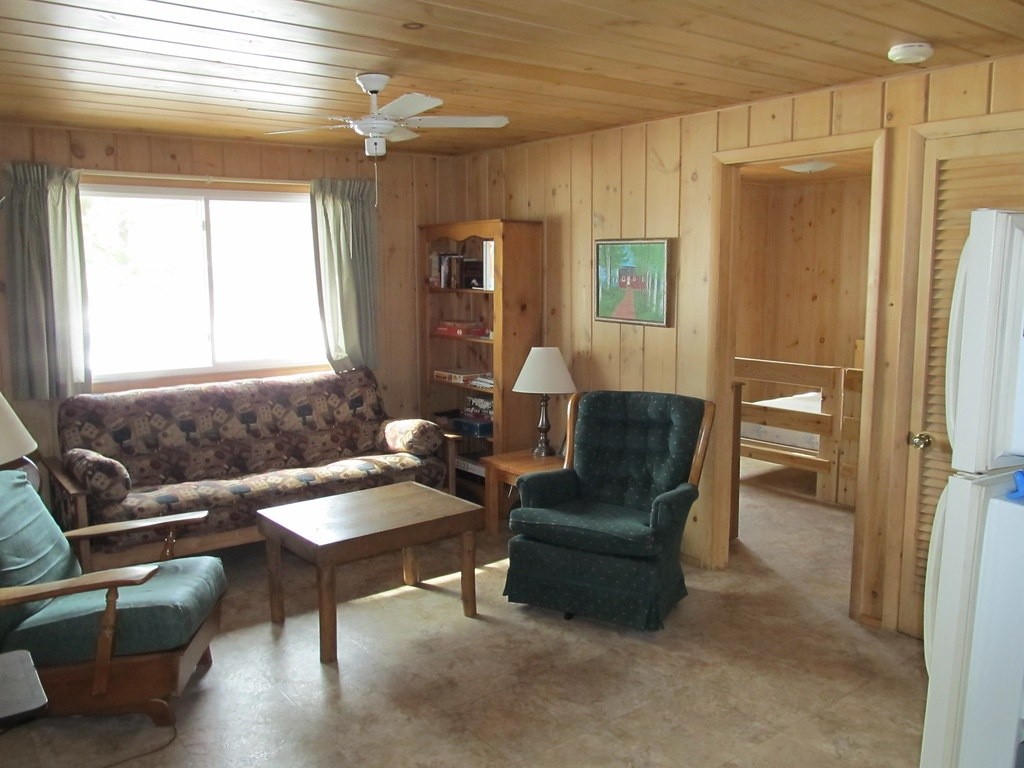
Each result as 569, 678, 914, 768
478, 451, 566, 536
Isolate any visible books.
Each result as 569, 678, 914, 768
429, 240, 495, 291
433, 319, 488, 337
432, 395, 493, 438
433, 368, 494, 388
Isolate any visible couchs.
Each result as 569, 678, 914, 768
1, 471, 226, 730
39, 366, 463, 574
503, 389, 715, 631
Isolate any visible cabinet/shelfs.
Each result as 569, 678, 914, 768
416, 220, 546, 507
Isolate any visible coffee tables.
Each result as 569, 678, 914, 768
254, 479, 483, 663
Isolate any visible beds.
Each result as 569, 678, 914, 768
732, 339, 864, 512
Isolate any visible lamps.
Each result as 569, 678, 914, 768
1, 393, 37, 471
362, 137, 388, 208
512, 345, 577, 456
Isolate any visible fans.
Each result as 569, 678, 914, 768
263, 74, 510, 142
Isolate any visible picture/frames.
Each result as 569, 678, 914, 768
591, 238, 670, 330
